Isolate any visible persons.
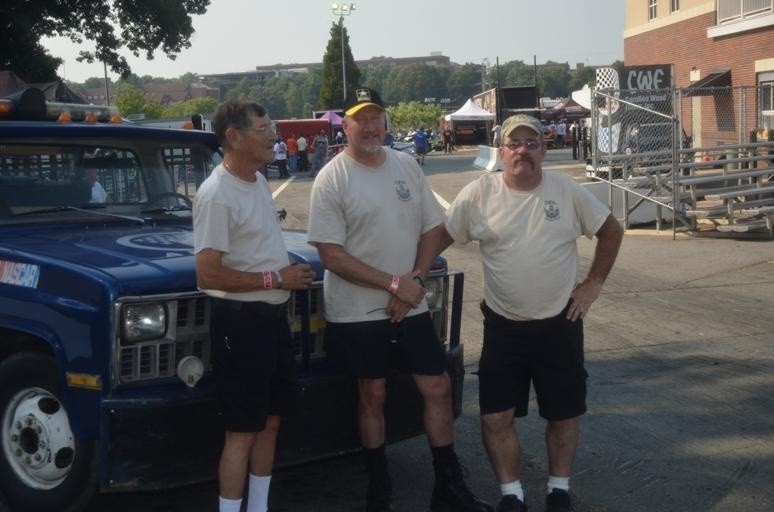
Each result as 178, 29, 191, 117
75, 166, 114, 206
415, 128, 427, 165
272, 138, 291, 179
335, 131, 344, 151
435, 114, 625, 512
491, 122, 502, 148
539, 118, 587, 149
190, 99, 317, 512
286, 132, 299, 173
309, 130, 328, 177
212, 141, 224, 167
319, 128, 329, 145
306, 87, 494, 512
383, 129, 394, 149
441, 126, 452, 154
295, 133, 309, 171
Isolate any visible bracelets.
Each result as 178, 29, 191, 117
274, 271, 282, 289
262, 270, 273, 289
387, 274, 400, 295
414, 274, 425, 289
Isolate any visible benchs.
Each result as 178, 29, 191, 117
592, 142, 773, 241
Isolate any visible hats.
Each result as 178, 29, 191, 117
502, 115, 544, 136
344, 87, 385, 116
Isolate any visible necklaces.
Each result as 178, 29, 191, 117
224, 162, 241, 179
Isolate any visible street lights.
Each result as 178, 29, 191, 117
330, 3, 356, 102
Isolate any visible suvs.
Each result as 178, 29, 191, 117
0, 121, 463, 511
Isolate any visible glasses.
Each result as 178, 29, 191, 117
235, 124, 276, 135
501, 140, 543, 149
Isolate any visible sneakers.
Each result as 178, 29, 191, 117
546, 488, 569, 512
496, 495, 528, 512
430, 484, 493, 512
